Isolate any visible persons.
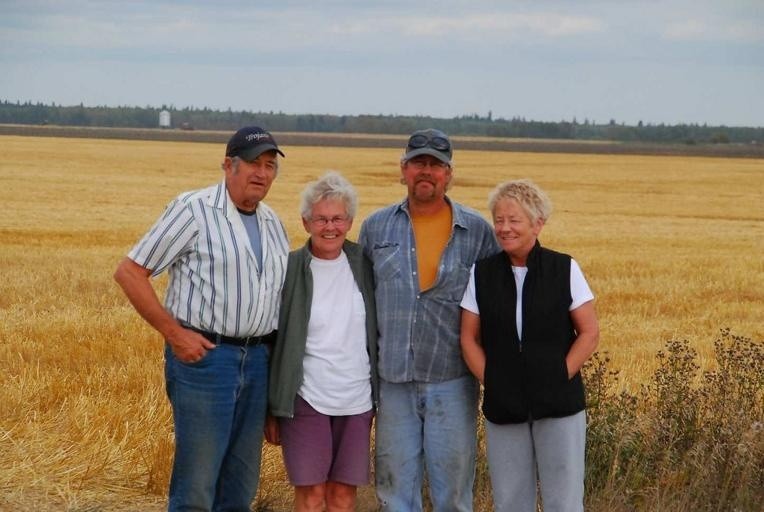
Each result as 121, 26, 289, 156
353, 127, 501, 510
264, 174, 380, 509
457, 178, 600, 511
112, 125, 292, 512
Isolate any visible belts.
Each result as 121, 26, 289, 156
180, 324, 272, 348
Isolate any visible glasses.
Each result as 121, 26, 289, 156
405, 134, 452, 157
310, 216, 349, 226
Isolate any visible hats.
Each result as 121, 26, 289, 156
404, 128, 452, 164
225, 126, 285, 163
